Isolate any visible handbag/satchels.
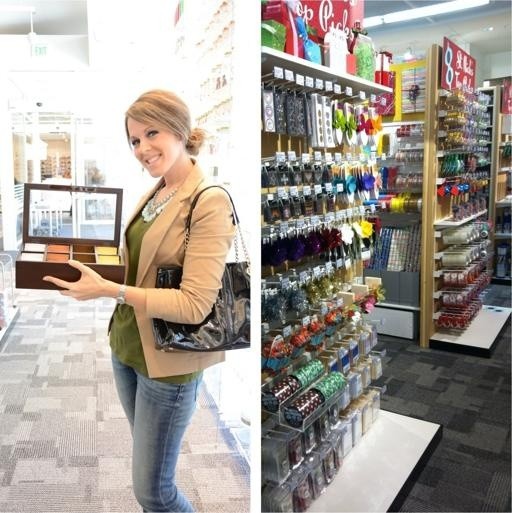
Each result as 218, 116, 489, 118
152, 185, 250, 352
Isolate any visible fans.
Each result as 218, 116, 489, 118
11, 12, 52, 55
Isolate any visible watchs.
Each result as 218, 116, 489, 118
117, 285, 125, 305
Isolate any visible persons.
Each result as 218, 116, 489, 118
42, 89, 236, 513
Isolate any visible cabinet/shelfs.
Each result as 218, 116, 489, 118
433, 88, 490, 331
261, 45, 442, 512
494, 103, 511, 277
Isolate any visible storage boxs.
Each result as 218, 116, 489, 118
16, 183, 127, 289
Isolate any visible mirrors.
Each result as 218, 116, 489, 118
28, 189, 117, 240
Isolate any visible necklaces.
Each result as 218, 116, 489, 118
148, 182, 178, 214
